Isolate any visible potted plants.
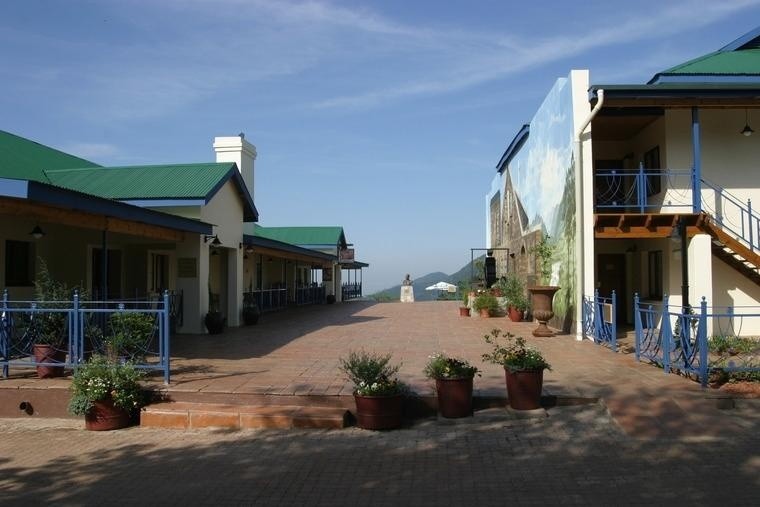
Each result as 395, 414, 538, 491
25, 251, 160, 432
242, 292, 261, 324
336, 329, 550, 432
459, 273, 527, 321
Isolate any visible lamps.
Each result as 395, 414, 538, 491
739, 107, 756, 138
239, 238, 254, 254
204, 233, 224, 248
26, 223, 48, 239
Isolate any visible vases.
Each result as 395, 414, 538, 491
205, 310, 226, 333
